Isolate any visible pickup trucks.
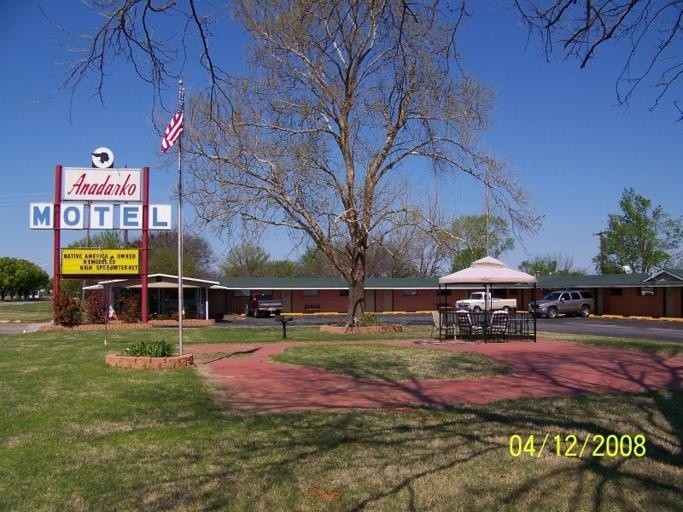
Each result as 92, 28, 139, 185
455, 292, 520, 314
242, 293, 284, 317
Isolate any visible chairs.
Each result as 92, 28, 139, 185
429, 309, 511, 345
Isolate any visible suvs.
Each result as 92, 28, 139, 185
528, 290, 596, 319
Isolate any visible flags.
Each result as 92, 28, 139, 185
159, 90, 184, 154
